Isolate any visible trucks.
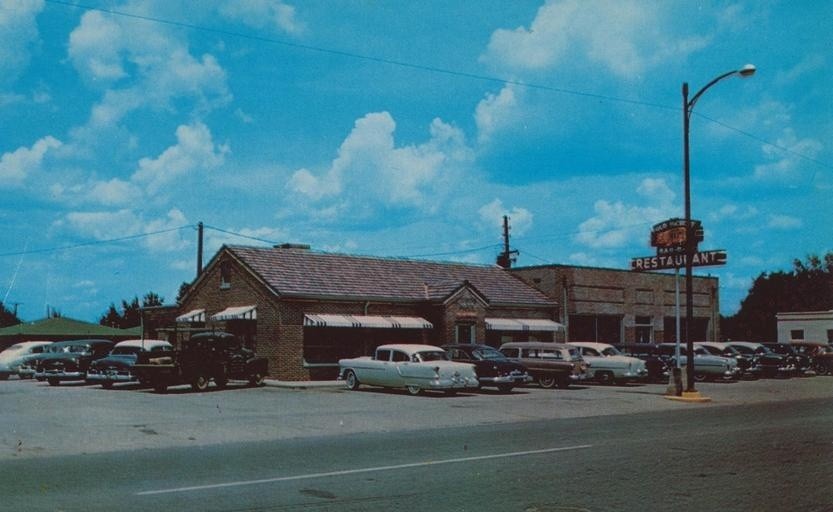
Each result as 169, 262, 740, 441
129, 332, 264, 393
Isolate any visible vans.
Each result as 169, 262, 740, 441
497, 341, 588, 390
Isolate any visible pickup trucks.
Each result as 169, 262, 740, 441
337, 343, 481, 400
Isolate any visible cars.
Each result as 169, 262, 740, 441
0, 339, 173, 386
613, 338, 833, 384
564, 341, 648, 387
439, 343, 529, 394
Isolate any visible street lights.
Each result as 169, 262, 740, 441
681, 65, 755, 402
